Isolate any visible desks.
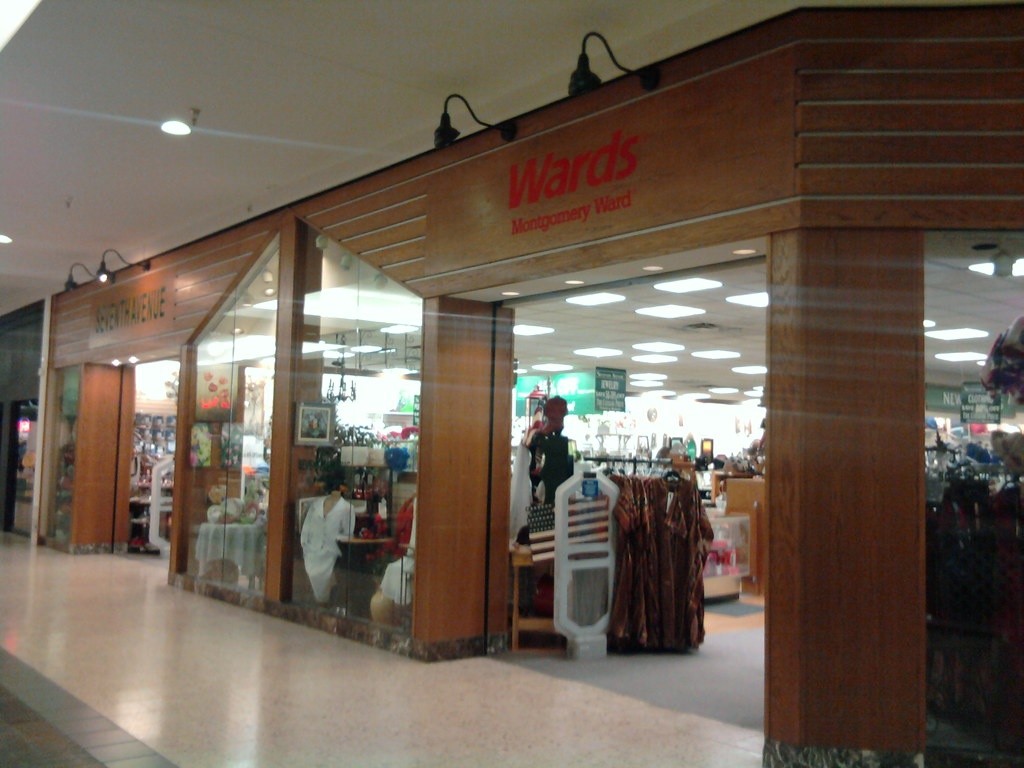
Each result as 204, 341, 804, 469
198, 522, 264, 589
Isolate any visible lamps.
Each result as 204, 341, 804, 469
95, 248, 151, 284
65, 263, 96, 293
433, 93, 517, 148
567, 31, 663, 95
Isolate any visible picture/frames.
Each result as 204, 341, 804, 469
294, 400, 335, 447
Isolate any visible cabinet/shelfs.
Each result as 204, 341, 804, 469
135, 400, 177, 459
702, 510, 751, 604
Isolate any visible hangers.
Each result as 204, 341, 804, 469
927, 462, 1024, 510
603, 455, 682, 482
